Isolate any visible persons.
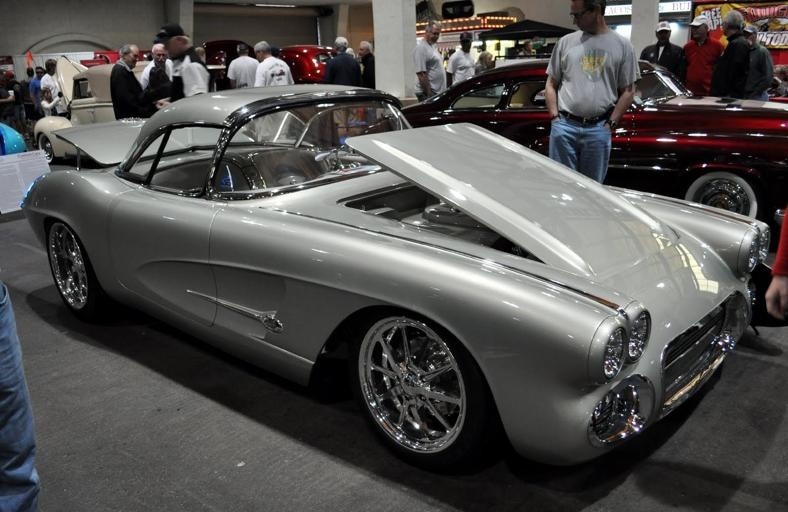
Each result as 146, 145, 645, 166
0, 271, 41, 511
327, 37, 375, 137
1, 59, 72, 148
545, 0, 635, 186
518, 41, 536, 59
228, 41, 294, 88
639, 12, 787, 101
110, 22, 211, 120
765, 204, 788, 320
414, 22, 492, 104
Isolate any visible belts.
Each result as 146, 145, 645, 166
557, 107, 614, 128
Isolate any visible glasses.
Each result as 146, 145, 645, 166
569, 9, 589, 20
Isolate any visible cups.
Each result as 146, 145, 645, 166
8, 91, 14, 96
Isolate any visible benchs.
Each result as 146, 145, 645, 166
149, 147, 325, 195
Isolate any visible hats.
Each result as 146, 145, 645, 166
154, 22, 187, 45
743, 25, 758, 36
654, 20, 672, 34
459, 32, 474, 43
688, 13, 710, 29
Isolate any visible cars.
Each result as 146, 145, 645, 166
0, 39, 262, 163
273, 45, 335, 83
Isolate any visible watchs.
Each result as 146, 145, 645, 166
606, 119, 617, 130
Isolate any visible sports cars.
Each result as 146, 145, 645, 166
16, 84, 769, 466
355, 59, 788, 219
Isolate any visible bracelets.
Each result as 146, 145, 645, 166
550, 115, 558, 121
